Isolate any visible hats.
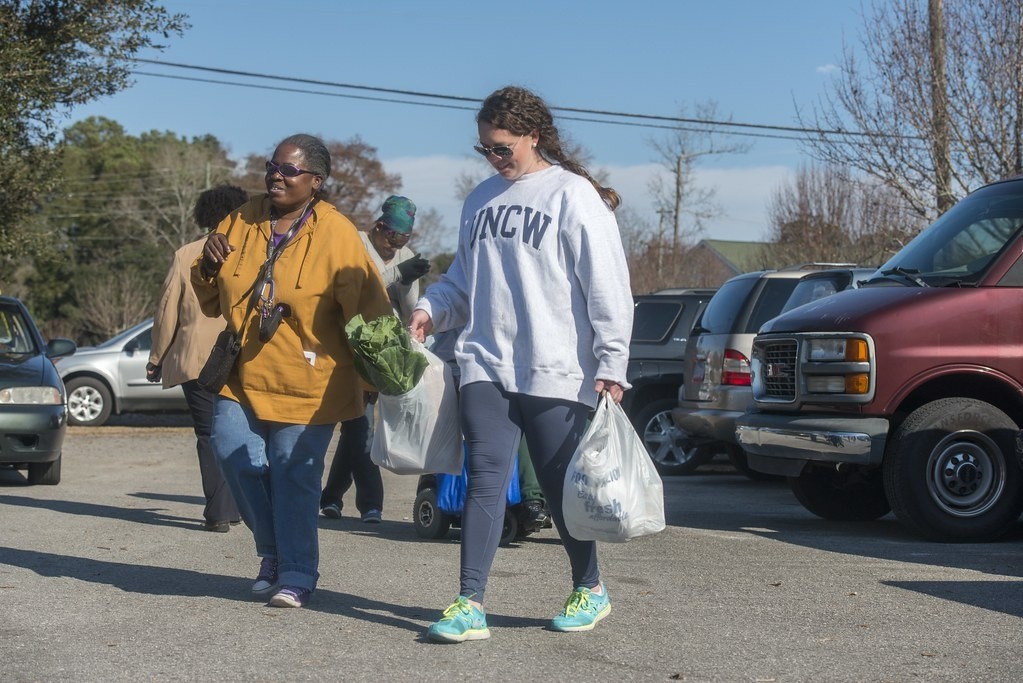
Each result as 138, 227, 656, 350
378, 196, 417, 236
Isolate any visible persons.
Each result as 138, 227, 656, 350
425, 325, 551, 531
146, 184, 250, 533
320, 195, 432, 523
407, 87, 635, 642
190, 134, 395, 607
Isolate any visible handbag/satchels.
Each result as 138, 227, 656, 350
371, 336, 464, 475
197, 332, 239, 392
562, 391, 665, 544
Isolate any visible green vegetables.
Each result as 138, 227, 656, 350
345, 313, 429, 397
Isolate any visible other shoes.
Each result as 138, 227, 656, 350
203, 517, 241, 533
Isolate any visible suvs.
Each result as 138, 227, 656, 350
626, 289, 724, 475
732, 173, 1023, 544
671, 262, 884, 480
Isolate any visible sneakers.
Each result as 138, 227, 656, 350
428, 595, 490, 642
269, 585, 309, 608
324, 504, 341, 518
251, 557, 278, 594
523, 504, 551, 530
551, 583, 611, 632
363, 508, 381, 523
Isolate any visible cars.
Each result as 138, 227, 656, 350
0, 294, 78, 485
54, 312, 192, 426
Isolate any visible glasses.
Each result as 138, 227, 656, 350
265, 161, 321, 177
474, 134, 524, 160
